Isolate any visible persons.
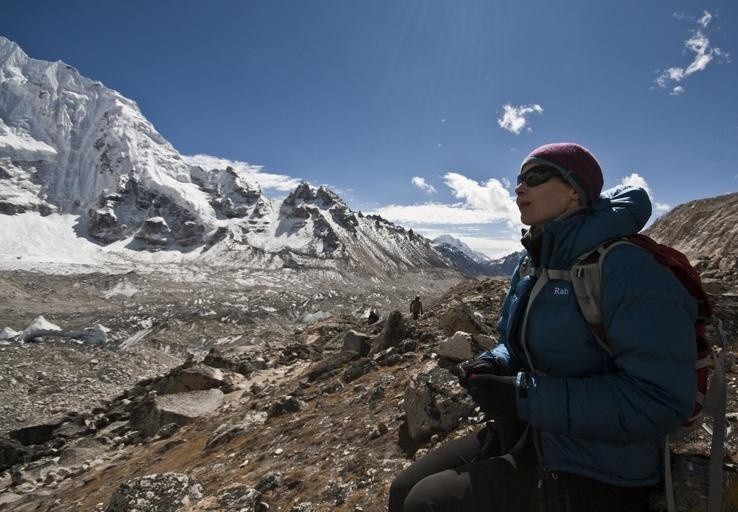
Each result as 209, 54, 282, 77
410, 293, 426, 320
390, 142, 697, 510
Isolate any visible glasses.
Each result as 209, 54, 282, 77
517, 165, 560, 187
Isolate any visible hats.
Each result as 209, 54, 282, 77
520, 143, 603, 205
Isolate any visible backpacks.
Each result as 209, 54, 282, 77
518, 234, 713, 428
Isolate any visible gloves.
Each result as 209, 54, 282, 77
456, 360, 495, 395
468, 374, 514, 416
